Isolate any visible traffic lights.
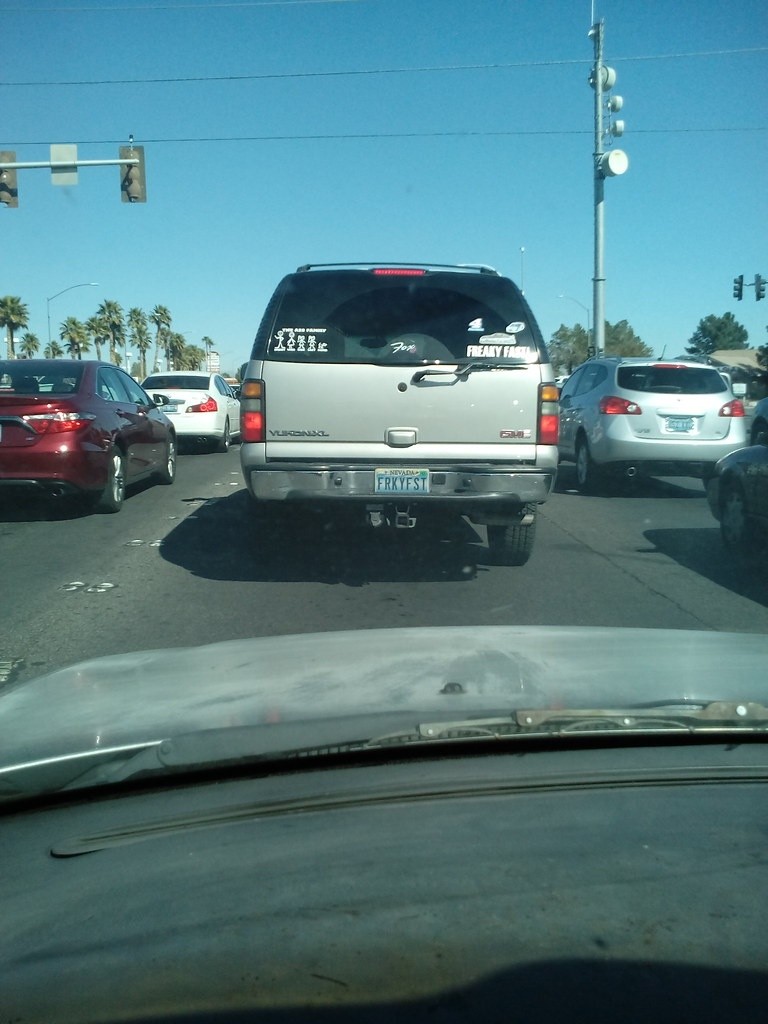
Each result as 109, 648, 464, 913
754, 274, 766, 301
734, 274, 743, 301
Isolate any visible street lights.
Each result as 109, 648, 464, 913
122, 313, 161, 367
46, 282, 99, 359
558, 294, 591, 350
519, 244, 526, 291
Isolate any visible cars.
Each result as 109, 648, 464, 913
0, 360, 177, 514
101, 369, 241, 453
705, 442, 768, 575
750, 397, 768, 446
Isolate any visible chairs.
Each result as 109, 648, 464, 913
15, 377, 39, 393
52, 383, 73, 392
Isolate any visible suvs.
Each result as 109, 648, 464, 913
235, 261, 560, 567
561, 353, 748, 495
